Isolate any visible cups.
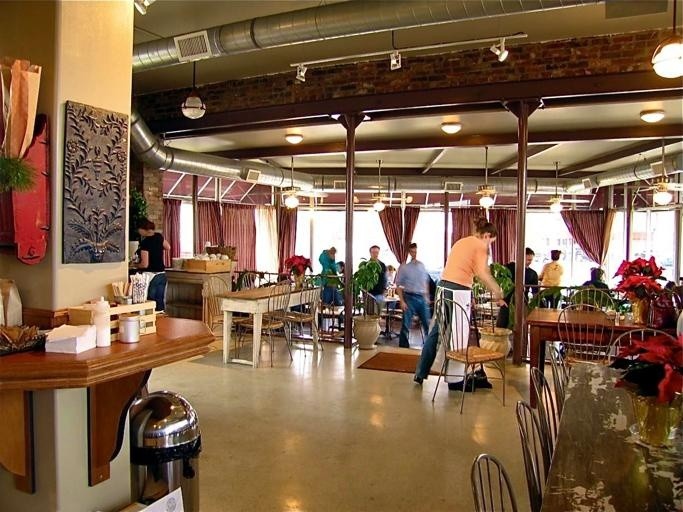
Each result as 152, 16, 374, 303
117, 313, 146, 344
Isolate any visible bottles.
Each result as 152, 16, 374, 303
93, 296, 111, 349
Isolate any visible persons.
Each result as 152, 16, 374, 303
383, 264, 396, 287
393, 243, 432, 348
413, 216, 508, 393
495, 246, 539, 328
362, 245, 387, 304
540, 250, 567, 309
334, 261, 346, 289
318, 246, 346, 323
128, 217, 171, 312
582, 265, 611, 297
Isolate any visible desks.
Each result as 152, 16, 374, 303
1, 313, 209, 495
161, 270, 230, 353
526, 304, 681, 411
542, 359, 683, 509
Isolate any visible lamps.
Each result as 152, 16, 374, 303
638, 0, 682, 207
180, 61, 208, 120
478, 146, 497, 210
374, 157, 385, 212
285, 133, 303, 146
284, 154, 300, 208
441, 123, 461, 135
296, 36, 509, 81
548, 160, 562, 213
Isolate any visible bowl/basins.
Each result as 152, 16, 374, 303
192, 252, 229, 261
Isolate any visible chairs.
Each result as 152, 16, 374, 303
558, 288, 616, 363
473, 339, 567, 512
432, 299, 507, 415
201, 272, 321, 368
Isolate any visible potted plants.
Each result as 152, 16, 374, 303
473, 259, 514, 367
348, 258, 383, 351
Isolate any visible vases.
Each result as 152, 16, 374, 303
634, 391, 673, 450
630, 296, 649, 324
294, 272, 304, 288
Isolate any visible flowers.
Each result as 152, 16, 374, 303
611, 335, 682, 400
612, 256, 664, 300
286, 254, 312, 275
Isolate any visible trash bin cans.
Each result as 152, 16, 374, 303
129, 390, 202, 512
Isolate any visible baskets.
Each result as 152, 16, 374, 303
206, 247, 237, 261
67, 299, 156, 343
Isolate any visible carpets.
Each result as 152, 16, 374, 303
356, 350, 447, 374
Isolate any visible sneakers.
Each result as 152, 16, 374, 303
414, 375, 423, 385
449, 384, 475, 392
